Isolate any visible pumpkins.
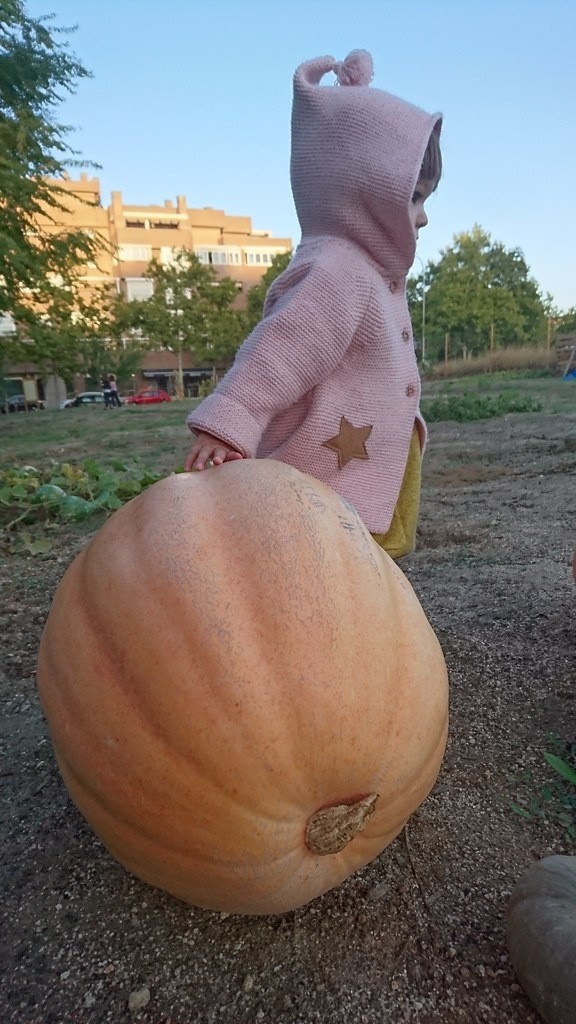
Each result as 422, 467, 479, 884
36, 464, 451, 915
508, 854, 576, 1024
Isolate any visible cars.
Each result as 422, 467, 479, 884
59, 390, 119, 410
0, 394, 46, 415
125, 389, 172, 404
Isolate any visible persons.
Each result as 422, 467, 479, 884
186, 82, 441, 559
100, 375, 122, 411
